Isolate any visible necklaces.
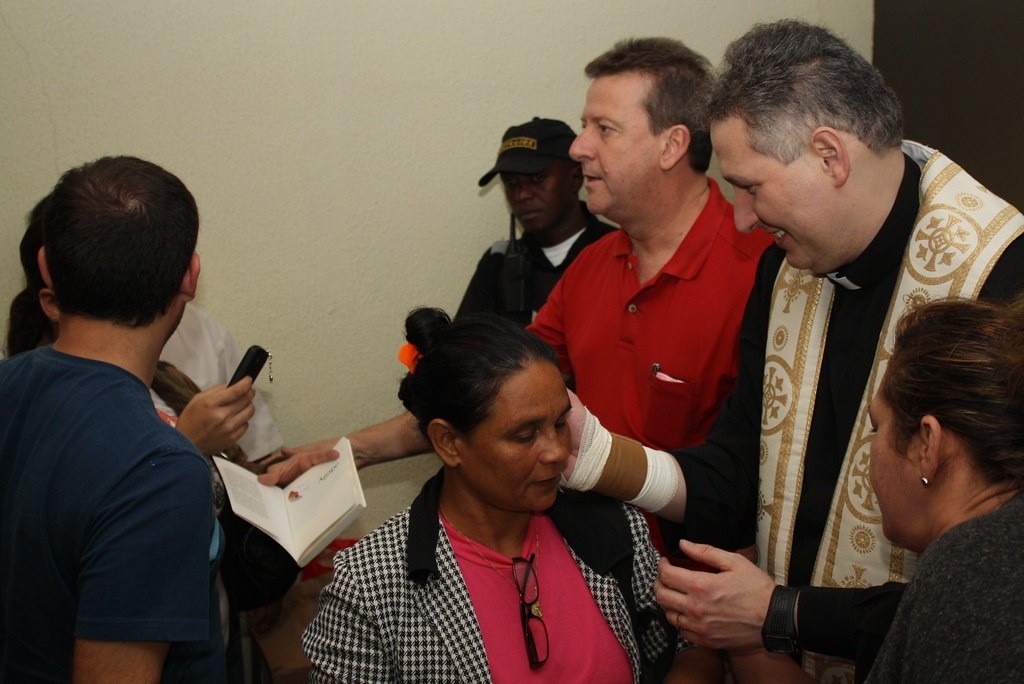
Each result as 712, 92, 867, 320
439, 502, 544, 617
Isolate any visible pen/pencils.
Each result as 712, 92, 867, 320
650, 363, 661, 375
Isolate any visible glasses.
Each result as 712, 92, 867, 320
512, 553, 549, 670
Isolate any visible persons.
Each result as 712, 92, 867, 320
301, 306, 681, 684
6, 187, 339, 647
862, 294, 1024, 684
0, 155, 229, 684
256, 38, 779, 552
279, 115, 621, 472
563, 18, 1023, 684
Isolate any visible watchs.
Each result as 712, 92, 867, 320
761, 585, 799, 655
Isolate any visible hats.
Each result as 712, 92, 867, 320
479, 117, 578, 186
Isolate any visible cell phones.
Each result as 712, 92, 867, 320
227, 346, 269, 388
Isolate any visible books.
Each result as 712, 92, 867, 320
213, 437, 367, 568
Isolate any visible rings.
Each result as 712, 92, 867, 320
676, 613, 682, 629
